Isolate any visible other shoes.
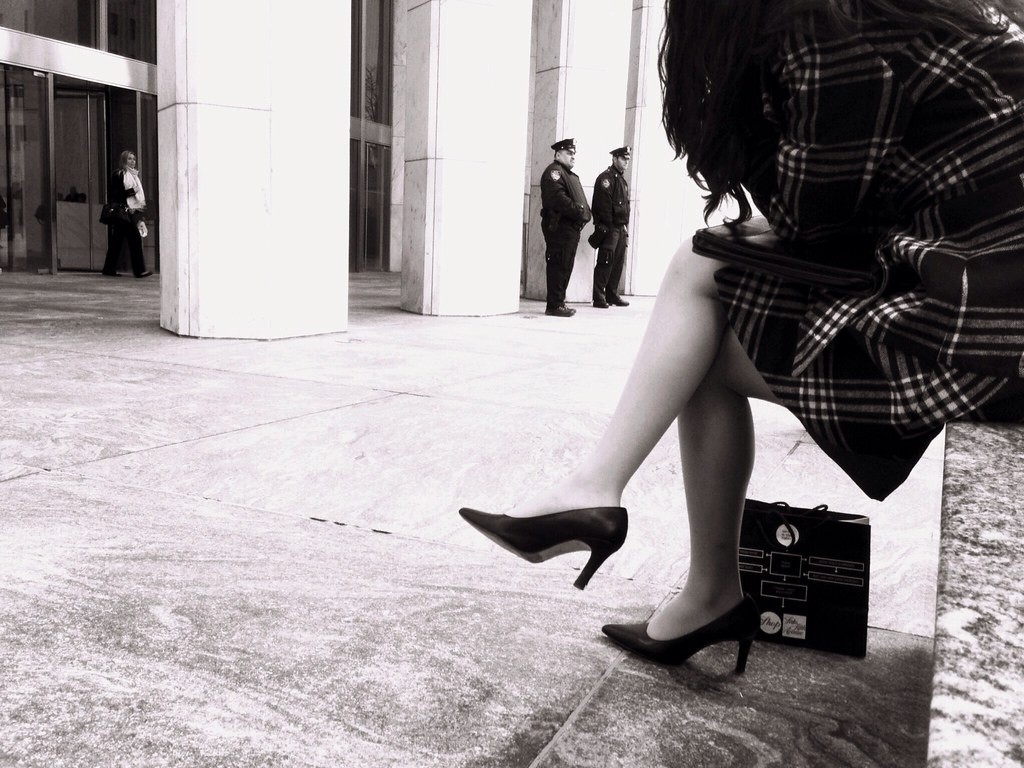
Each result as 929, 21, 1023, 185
136, 271, 153, 278
110, 274, 122, 276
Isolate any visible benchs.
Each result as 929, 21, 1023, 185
925, 412, 1023, 768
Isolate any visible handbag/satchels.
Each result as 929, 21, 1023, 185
693, 217, 882, 297
98, 201, 132, 225
736, 499, 872, 658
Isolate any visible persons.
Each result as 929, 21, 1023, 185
101, 150, 153, 279
1, 194, 7, 250
458, 0, 1024, 672
62, 185, 87, 204
540, 138, 592, 317
590, 145, 632, 309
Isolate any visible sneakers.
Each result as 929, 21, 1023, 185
545, 304, 577, 317
593, 301, 609, 308
607, 299, 629, 306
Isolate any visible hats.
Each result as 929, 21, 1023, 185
550, 138, 576, 153
609, 145, 632, 159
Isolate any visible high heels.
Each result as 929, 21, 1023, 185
459, 506, 629, 590
601, 592, 762, 673
